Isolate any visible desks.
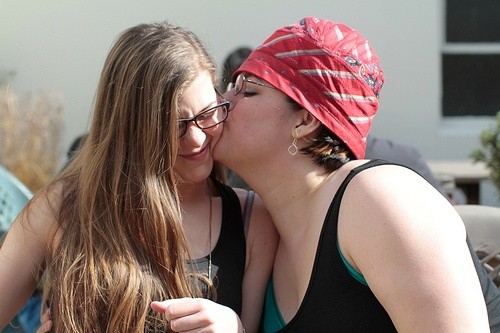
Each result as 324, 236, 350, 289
426, 160, 494, 205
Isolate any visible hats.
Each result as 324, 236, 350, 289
230, 16, 384, 160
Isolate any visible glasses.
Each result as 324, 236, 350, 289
227, 74, 276, 96
176, 87, 229, 138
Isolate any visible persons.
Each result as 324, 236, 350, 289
0, 21, 280, 333
33, 17, 500, 333
67, 47, 441, 192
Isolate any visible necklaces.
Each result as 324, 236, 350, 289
200, 181, 213, 299
